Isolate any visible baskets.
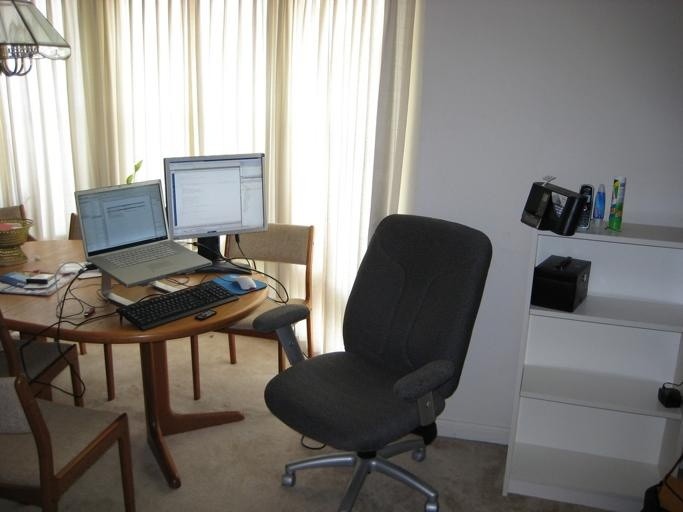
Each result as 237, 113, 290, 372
0, 218, 33, 246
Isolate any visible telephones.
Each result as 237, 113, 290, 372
576, 183, 595, 229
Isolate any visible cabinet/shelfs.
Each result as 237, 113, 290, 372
499, 215, 683, 512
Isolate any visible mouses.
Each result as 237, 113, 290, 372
232, 276, 256, 291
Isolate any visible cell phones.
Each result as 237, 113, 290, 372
195, 310, 216, 321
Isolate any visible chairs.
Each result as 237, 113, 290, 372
16, 338, 86, 408
186, 220, 326, 404
1, 203, 39, 245
0, 308, 145, 511
251, 211, 494, 512
43, 210, 120, 405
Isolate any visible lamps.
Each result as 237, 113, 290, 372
1, 1, 75, 82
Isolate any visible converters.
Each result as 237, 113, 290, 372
658, 387, 681, 408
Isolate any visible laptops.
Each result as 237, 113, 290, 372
74, 179, 213, 288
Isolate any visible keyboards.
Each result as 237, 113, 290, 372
115, 280, 240, 331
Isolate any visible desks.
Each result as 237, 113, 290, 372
0, 230, 268, 493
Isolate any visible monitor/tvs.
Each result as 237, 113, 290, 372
163, 153, 268, 274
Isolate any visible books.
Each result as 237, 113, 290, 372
0, 273, 74, 296
